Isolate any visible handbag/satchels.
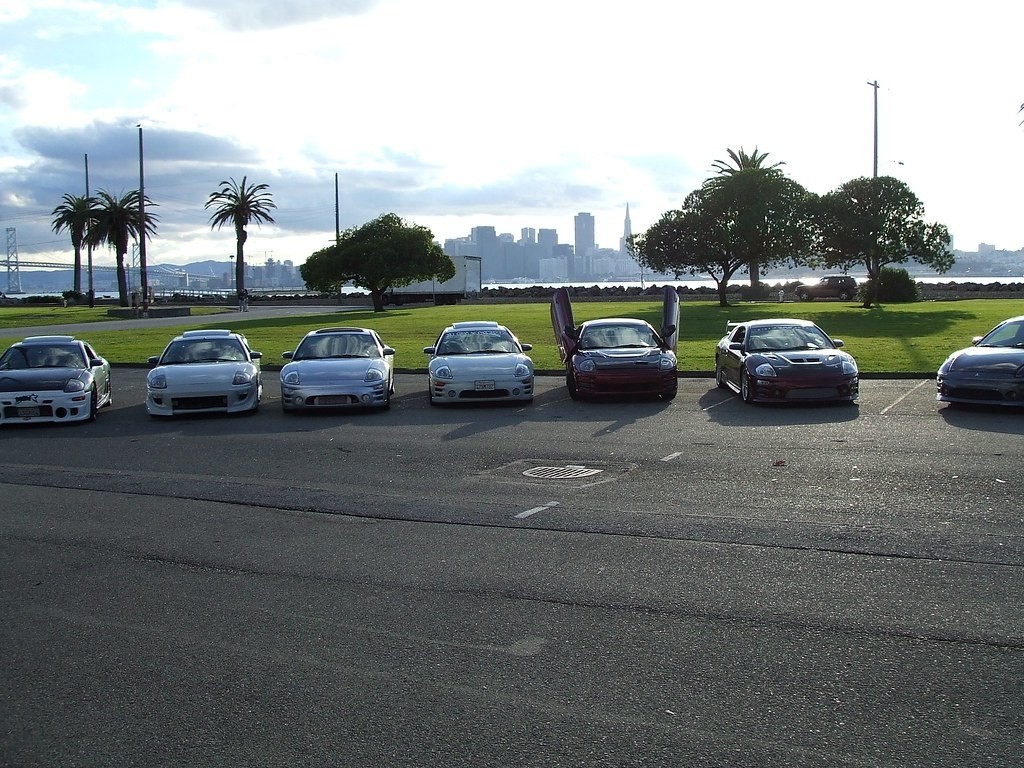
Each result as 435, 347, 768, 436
246, 299, 249, 303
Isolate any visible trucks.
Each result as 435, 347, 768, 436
382, 256, 482, 307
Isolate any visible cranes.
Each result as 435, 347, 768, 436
251, 250, 273, 262
244, 254, 253, 266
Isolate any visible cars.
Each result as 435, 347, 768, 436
714, 318, 859, 405
550, 285, 681, 399
145, 329, 262, 417
278, 326, 395, 414
424, 322, 536, 407
936, 315, 1024, 408
0, 335, 113, 425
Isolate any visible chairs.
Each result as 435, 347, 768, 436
7, 352, 30, 369
444, 342, 464, 353
44, 352, 74, 367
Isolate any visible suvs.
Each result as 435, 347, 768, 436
795, 275, 858, 302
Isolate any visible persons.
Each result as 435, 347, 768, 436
238, 289, 249, 312
779, 289, 784, 302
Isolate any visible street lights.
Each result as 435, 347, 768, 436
873, 159, 904, 277
230, 256, 234, 294
